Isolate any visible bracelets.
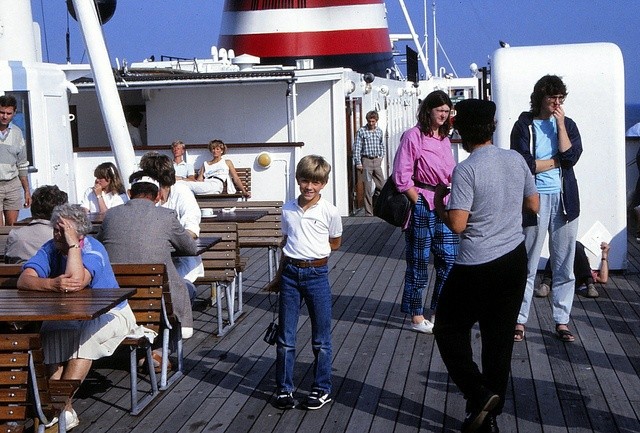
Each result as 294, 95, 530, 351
601, 258, 608, 261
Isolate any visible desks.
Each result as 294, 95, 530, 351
0, 287, 139, 433
88, 211, 269, 223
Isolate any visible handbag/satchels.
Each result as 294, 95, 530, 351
373, 173, 410, 227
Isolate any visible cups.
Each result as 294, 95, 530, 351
201, 207, 214, 216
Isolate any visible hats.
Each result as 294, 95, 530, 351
455, 99, 495, 124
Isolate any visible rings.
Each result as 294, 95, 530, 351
64, 287, 68, 293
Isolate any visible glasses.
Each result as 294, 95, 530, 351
451, 115, 458, 130
545, 96, 565, 102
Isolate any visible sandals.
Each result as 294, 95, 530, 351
514, 323, 525, 342
555, 323, 574, 341
146, 353, 173, 373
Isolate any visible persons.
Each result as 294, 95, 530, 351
127, 111, 143, 146
267, 156, 343, 411
4, 185, 67, 265
352, 111, 385, 217
393, 91, 460, 335
171, 140, 195, 181
434, 99, 540, 433
95, 170, 198, 373
17, 203, 137, 432
79, 162, 130, 213
184, 140, 251, 198
0, 96, 32, 226
510, 75, 582, 342
140, 152, 202, 284
534, 240, 610, 297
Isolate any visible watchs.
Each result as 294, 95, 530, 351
96, 195, 102, 199
68, 244, 80, 249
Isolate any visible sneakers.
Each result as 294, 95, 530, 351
535, 283, 549, 297
45, 408, 80, 432
460, 391, 500, 433
276, 390, 295, 409
410, 319, 434, 334
481, 416, 499, 432
587, 283, 599, 298
181, 326, 193, 339
301, 389, 331, 410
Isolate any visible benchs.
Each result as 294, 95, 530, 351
0, 335, 31, 433
198, 200, 284, 285
0, 264, 165, 416
190, 169, 252, 208
159, 266, 184, 392
1, 222, 238, 337
27, 333, 82, 432
233, 224, 250, 323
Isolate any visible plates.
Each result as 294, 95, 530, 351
202, 214, 218, 217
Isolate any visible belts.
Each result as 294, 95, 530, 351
362, 155, 377, 159
413, 180, 436, 192
285, 257, 327, 268
208, 176, 224, 182
